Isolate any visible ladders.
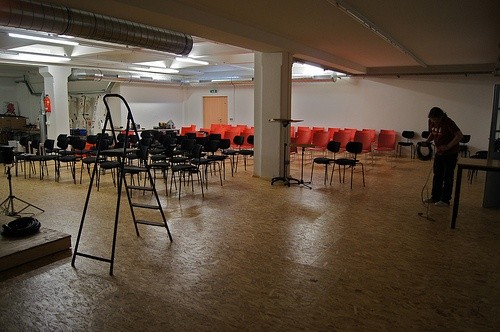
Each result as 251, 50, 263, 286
71, 94, 173, 276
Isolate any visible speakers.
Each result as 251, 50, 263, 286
0, 146, 14, 164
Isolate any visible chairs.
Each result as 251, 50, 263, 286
457, 133, 488, 184
10, 123, 435, 201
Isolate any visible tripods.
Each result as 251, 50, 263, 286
284, 144, 315, 189
0, 164, 45, 216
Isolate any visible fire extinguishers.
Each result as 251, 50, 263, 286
44, 94, 51, 113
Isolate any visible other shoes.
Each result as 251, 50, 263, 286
434, 201, 449, 207
424, 197, 435, 203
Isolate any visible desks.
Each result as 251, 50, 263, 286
449, 158, 500, 228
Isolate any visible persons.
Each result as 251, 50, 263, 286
424, 107, 464, 207
